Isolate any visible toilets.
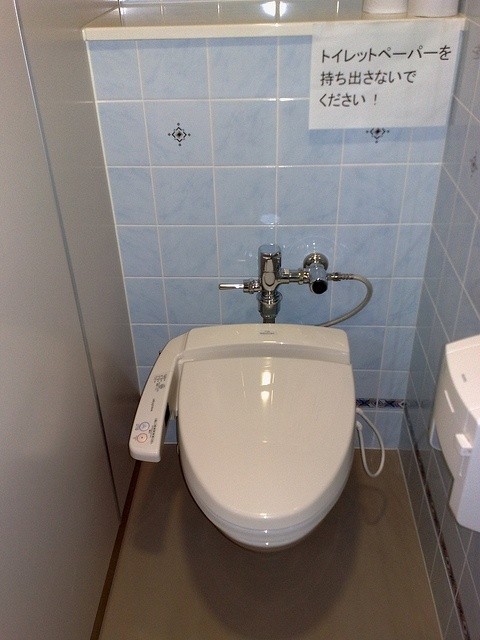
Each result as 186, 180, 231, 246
128, 323, 357, 555
